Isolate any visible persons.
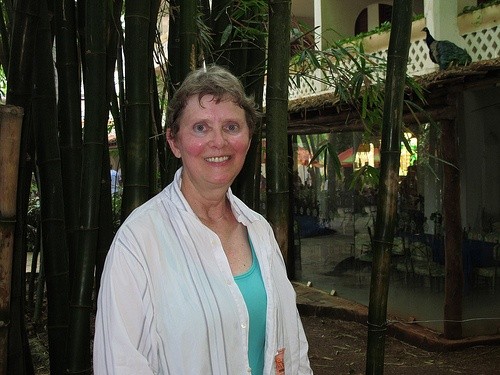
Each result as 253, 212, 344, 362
93, 64, 314, 374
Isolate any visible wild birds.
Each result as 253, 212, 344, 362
420, 27, 472, 71
